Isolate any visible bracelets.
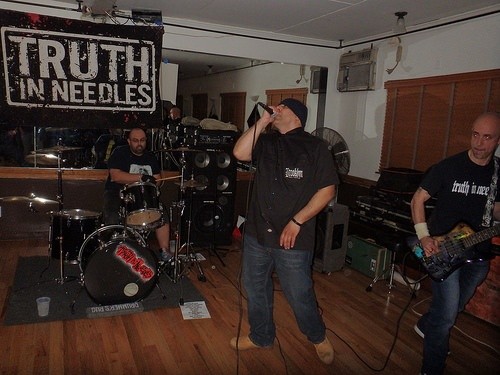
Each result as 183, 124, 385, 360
414, 222, 430, 238
292, 217, 302, 227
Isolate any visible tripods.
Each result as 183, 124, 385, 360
159, 153, 206, 305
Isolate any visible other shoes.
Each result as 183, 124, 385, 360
159, 248, 174, 261
313, 334, 335, 366
230, 336, 259, 351
413, 317, 451, 355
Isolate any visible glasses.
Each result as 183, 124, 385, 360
131, 137, 148, 144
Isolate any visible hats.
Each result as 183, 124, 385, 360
280, 98, 307, 129
103, 113, 130, 130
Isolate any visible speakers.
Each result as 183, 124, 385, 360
171, 144, 238, 247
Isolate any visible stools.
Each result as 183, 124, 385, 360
366, 232, 417, 300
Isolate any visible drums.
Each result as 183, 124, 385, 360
76, 224, 157, 307
49, 208, 104, 265
120, 184, 166, 229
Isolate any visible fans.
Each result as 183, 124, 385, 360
311, 127, 351, 207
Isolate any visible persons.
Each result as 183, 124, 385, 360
92, 129, 128, 169
167, 107, 183, 170
105, 128, 174, 261
231, 98, 341, 364
411, 112, 500, 375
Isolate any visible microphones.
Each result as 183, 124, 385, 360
258, 102, 273, 114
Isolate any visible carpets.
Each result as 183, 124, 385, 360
3, 255, 207, 325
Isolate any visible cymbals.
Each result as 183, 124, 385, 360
32, 145, 82, 152
175, 179, 209, 186
25, 153, 66, 164
0, 196, 60, 203
46, 127, 72, 131
160, 147, 202, 152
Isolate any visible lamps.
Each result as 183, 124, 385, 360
250, 95, 259, 101
395, 11, 408, 33
209, 96, 216, 102
207, 65, 213, 75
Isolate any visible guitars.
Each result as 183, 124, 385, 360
402, 216, 500, 283
